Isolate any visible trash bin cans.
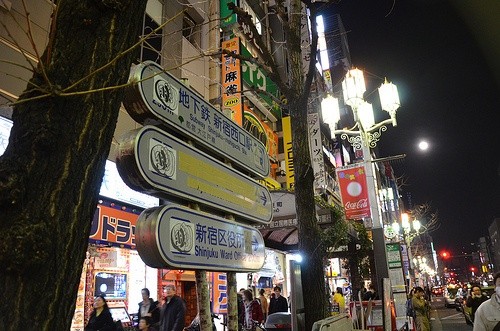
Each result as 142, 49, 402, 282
265, 312, 292, 331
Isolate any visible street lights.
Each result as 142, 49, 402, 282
392, 212, 421, 288
320, 66, 402, 331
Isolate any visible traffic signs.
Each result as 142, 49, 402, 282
134, 203, 266, 274
116, 123, 274, 226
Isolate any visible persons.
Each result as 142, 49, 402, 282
406, 285, 432, 331
331, 287, 345, 313
137, 285, 186, 331
86, 295, 114, 331
466, 286, 490, 322
237, 286, 291, 331
456, 282, 471, 306
473, 272, 500, 331
355, 285, 380, 300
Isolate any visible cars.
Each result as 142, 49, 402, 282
432, 285, 495, 327
